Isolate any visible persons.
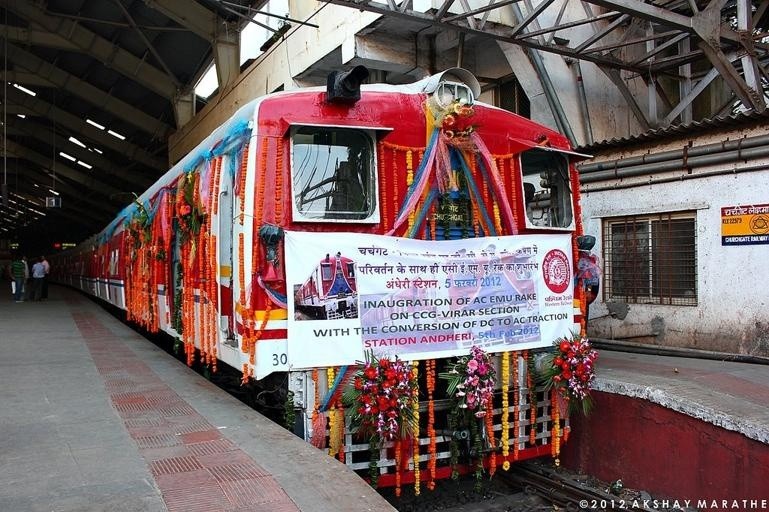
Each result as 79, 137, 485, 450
524, 182, 536, 211
4, 252, 51, 304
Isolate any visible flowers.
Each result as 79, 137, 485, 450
175, 183, 192, 232
448, 345, 497, 418
441, 92, 481, 148
352, 356, 414, 443
554, 335, 599, 402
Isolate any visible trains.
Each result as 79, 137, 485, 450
30, 67, 601, 490
